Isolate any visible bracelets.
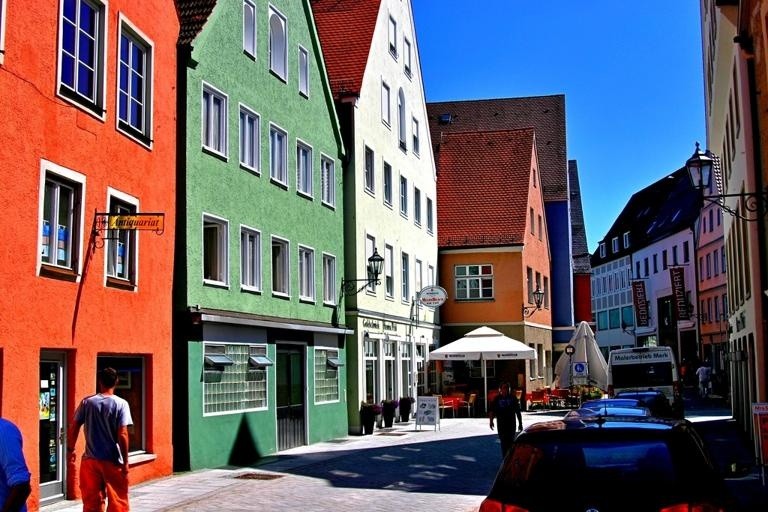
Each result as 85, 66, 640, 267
65, 446, 76, 452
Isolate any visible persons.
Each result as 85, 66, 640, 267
0, 417, 32, 512
489, 382, 524, 460
64, 368, 134, 512
696, 362, 711, 398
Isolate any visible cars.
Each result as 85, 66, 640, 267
477, 389, 753, 511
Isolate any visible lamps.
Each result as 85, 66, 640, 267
339, 247, 385, 306
521, 281, 544, 319
687, 301, 707, 321
685, 141, 768, 222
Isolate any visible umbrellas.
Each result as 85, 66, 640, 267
555, 321, 608, 406
429, 325, 537, 414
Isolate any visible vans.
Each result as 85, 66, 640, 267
606, 345, 685, 419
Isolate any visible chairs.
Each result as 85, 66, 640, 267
433, 384, 570, 419
